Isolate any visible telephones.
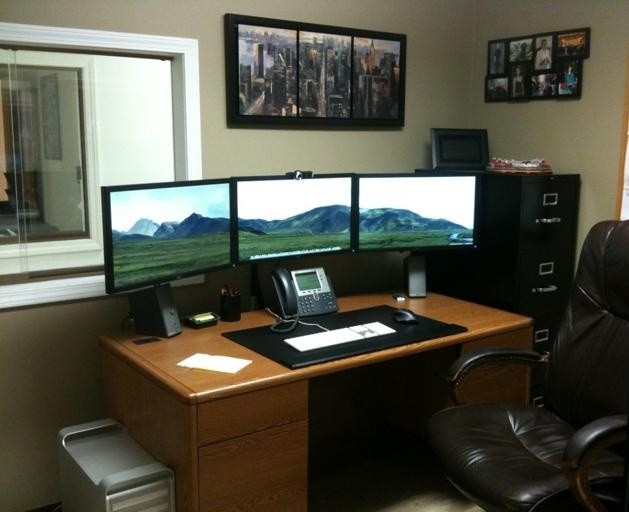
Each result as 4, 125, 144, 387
271, 267, 340, 319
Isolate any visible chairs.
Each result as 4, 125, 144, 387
420, 214, 629, 512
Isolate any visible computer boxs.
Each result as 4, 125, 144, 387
58, 417, 175, 512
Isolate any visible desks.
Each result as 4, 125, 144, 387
92, 281, 539, 511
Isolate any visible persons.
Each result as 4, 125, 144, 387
562, 64, 577, 86
536, 39, 552, 68
513, 67, 525, 98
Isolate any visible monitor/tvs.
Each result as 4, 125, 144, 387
355, 172, 480, 254
231, 173, 356, 268
101, 178, 235, 294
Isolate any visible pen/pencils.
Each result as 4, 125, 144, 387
219, 281, 242, 296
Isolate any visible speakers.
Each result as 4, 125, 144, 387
404, 256, 427, 298
129, 283, 184, 338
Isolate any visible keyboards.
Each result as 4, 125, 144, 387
284, 321, 397, 353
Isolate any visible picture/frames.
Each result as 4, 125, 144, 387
221, 12, 409, 130
425, 124, 494, 173
476, 23, 593, 103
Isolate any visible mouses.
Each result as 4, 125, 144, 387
392, 309, 418, 322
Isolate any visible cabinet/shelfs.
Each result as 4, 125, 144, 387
467, 167, 583, 413
